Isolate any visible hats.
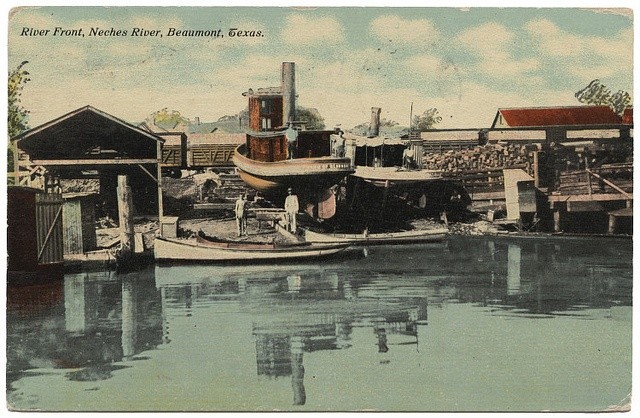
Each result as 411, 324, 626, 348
287, 187, 292, 192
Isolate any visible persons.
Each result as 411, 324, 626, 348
402, 143, 414, 170
284, 188, 299, 234
364, 227, 370, 239
285, 123, 299, 160
450, 190, 461, 200
235, 193, 248, 236
332, 131, 346, 159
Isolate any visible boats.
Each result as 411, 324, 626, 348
232, 60, 355, 209
